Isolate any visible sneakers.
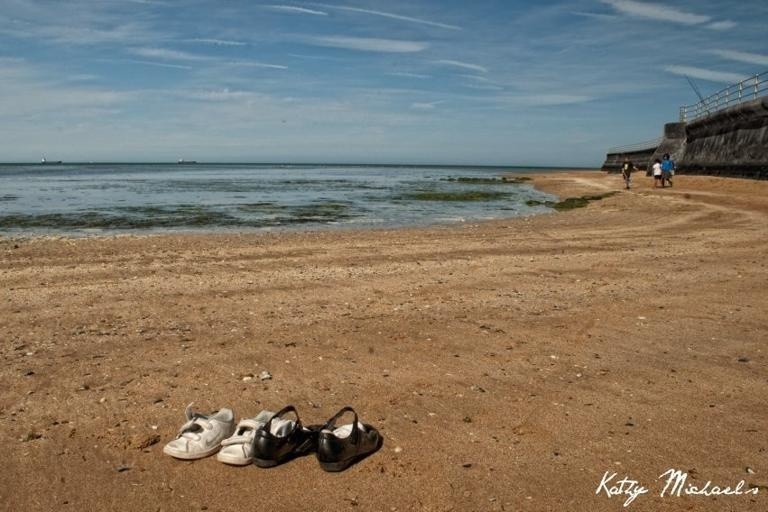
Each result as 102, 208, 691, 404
163, 401, 235, 461
217, 410, 275, 467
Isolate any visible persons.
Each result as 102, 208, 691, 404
622, 157, 639, 189
651, 158, 663, 186
660, 154, 674, 188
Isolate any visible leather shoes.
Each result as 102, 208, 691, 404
316, 406, 380, 473
252, 406, 337, 470
